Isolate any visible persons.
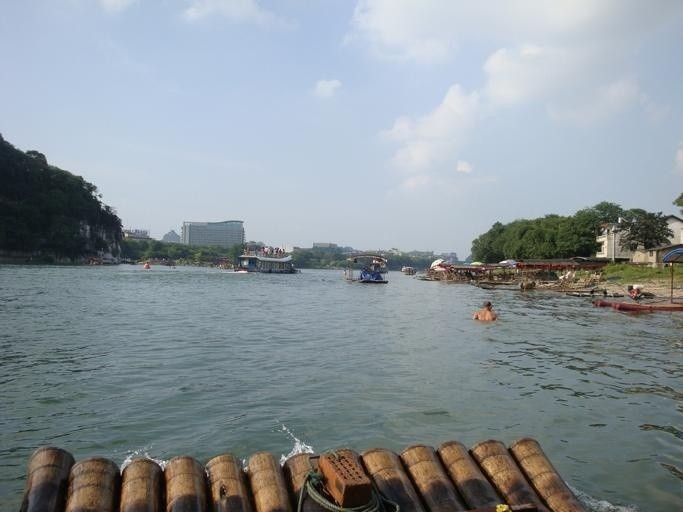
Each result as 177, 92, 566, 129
242, 245, 286, 258
144, 262, 151, 269
472, 301, 497, 322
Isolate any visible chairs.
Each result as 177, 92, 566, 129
561, 272, 602, 288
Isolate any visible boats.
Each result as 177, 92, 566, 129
402, 265, 416, 275
233, 254, 301, 273
344, 255, 389, 283
